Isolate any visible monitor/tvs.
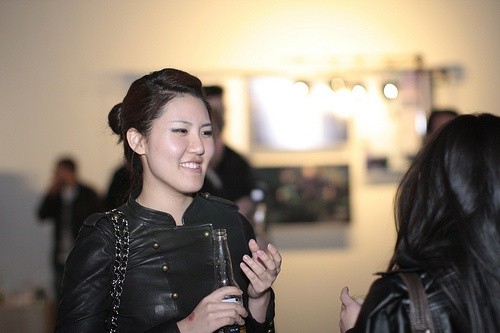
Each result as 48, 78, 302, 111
258, 163, 355, 228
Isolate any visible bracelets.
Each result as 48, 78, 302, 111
248, 289, 269, 301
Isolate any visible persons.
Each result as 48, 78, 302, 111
340, 114, 500, 333
423, 110, 457, 144
107, 164, 143, 211
38, 159, 99, 289
199, 85, 254, 221
56, 68, 282, 333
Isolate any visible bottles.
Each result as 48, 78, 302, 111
211, 229, 246, 333
